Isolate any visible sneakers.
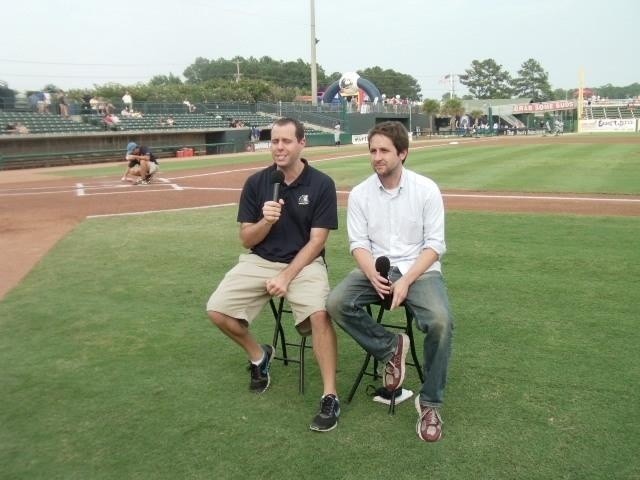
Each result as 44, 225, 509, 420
310, 394, 340, 432
385, 333, 410, 391
249, 344, 275, 395
415, 394, 443, 442
134, 174, 152, 184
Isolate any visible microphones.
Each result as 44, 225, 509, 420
376, 256, 390, 310
271, 170, 285, 203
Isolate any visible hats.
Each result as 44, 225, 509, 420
127, 142, 137, 154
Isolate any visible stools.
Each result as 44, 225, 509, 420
268, 247, 326, 396
347, 298, 425, 416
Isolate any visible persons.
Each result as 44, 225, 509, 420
332, 121, 340, 146
5, 89, 260, 141
326, 120, 455, 442
206, 115, 340, 432
121, 142, 158, 185
382, 96, 563, 136
